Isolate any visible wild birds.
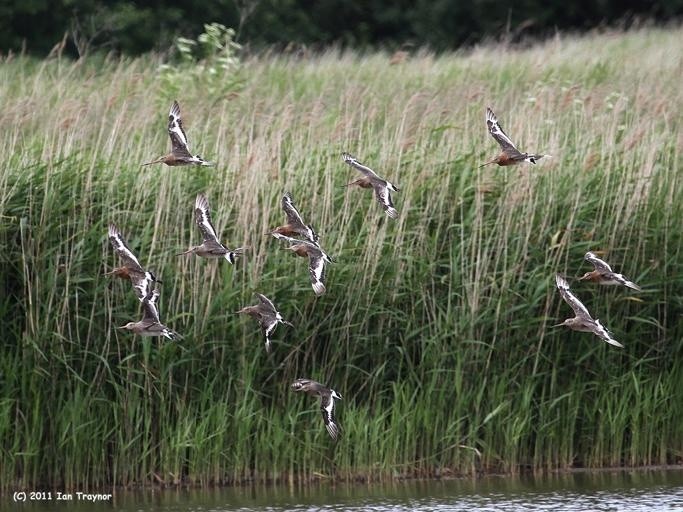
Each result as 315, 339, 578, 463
102, 219, 186, 344
577, 250, 642, 292
339, 152, 401, 221
174, 192, 237, 266
140, 100, 216, 167
263, 191, 334, 296
478, 107, 545, 168
234, 292, 296, 356
550, 272, 626, 349
290, 378, 345, 443
